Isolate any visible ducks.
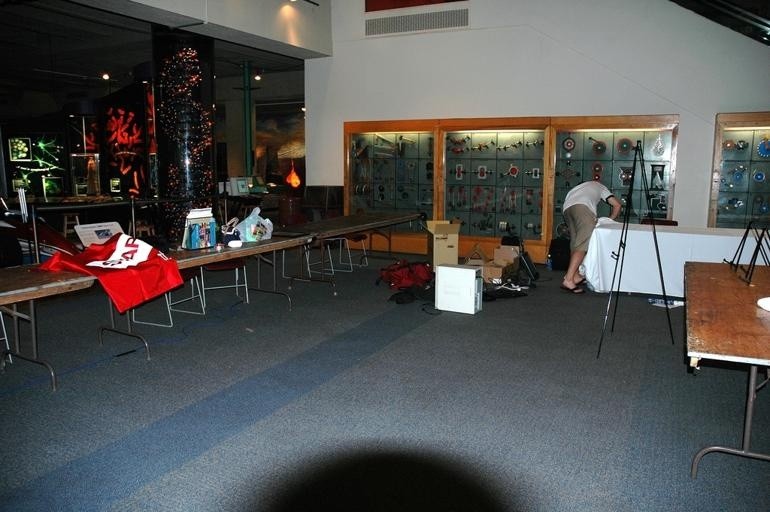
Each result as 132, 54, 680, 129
520, 251, 538, 281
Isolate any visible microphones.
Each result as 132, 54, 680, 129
129, 219, 155, 238
62, 210, 80, 237
199, 259, 253, 306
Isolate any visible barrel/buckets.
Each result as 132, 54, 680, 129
559, 285, 585, 296
575, 278, 588, 288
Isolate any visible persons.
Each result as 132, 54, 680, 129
560, 181, 621, 294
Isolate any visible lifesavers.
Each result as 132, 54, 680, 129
476, 276, 482, 313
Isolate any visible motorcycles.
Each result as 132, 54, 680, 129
596, 141, 676, 357
724, 213, 766, 271
743, 225, 766, 285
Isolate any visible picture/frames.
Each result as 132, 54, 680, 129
91, 235, 314, 361
684, 261, 770, 479
282, 209, 424, 284
1, 260, 152, 388
215, 195, 285, 229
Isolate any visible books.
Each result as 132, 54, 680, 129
426, 219, 460, 273
466, 259, 512, 284
494, 245, 520, 271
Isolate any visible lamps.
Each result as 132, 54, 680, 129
276, 209, 335, 282
132, 235, 207, 328
322, 206, 370, 273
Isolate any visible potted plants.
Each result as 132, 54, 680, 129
7, 136, 32, 162
70, 152, 102, 197
41, 175, 66, 203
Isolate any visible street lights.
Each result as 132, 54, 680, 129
220, 217, 241, 247
373, 257, 434, 290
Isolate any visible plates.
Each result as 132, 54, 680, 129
707, 111, 770, 229
437, 115, 550, 264
342, 120, 439, 256
548, 114, 681, 257
